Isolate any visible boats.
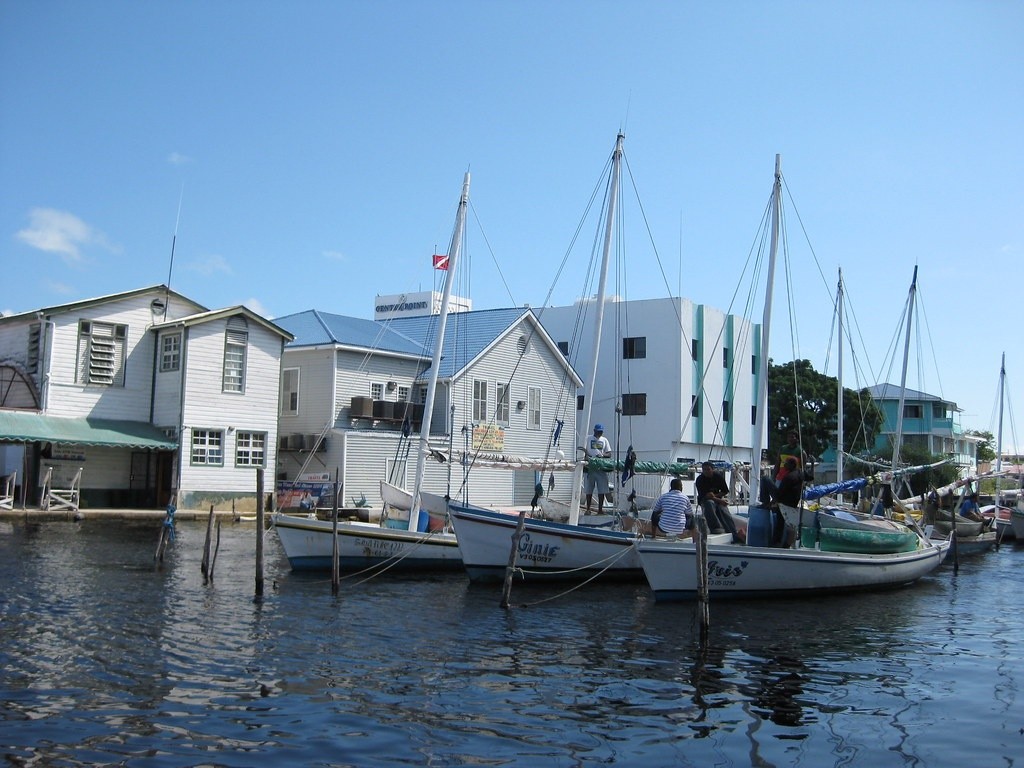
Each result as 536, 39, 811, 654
384, 517, 411, 531
418, 489, 498, 522
797, 525, 916, 555
536, 495, 599, 520
387, 504, 409, 521
378, 481, 414, 511
777, 501, 905, 533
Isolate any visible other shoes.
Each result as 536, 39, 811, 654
734, 536, 744, 547
757, 502, 769, 508
712, 528, 725, 534
584, 509, 592, 515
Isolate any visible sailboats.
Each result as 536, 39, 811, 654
443, 125, 735, 587
626, 152, 956, 603
610, 259, 1024, 557
267, 163, 659, 577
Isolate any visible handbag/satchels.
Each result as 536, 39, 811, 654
805, 455, 815, 481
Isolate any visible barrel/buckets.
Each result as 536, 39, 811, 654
746, 505, 773, 548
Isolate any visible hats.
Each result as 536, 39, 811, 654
781, 456, 798, 465
594, 424, 603, 431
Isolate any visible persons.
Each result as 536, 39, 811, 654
758, 431, 814, 547
584, 424, 612, 515
960, 494, 992, 527
651, 479, 697, 544
696, 462, 739, 543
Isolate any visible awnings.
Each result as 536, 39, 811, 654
0, 412, 179, 450
